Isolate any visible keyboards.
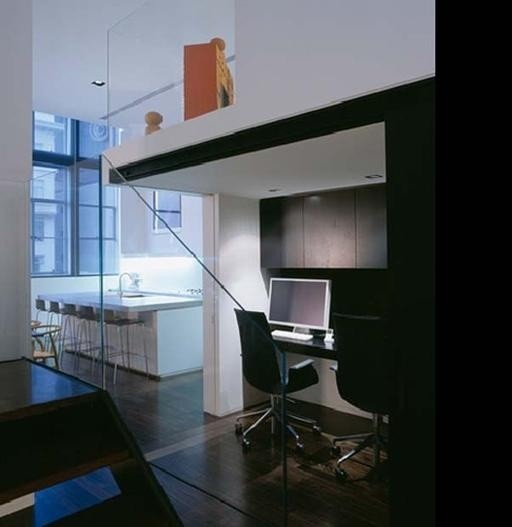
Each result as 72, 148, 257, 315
271, 330, 313, 341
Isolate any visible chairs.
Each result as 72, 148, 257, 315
233, 308, 320, 452
31, 312, 62, 371
331, 310, 394, 476
35, 298, 152, 386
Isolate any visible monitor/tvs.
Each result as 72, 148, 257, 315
267, 278, 331, 333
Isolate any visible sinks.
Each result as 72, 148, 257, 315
125, 295, 150, 298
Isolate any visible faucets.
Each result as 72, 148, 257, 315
118, 273, 133, 296
